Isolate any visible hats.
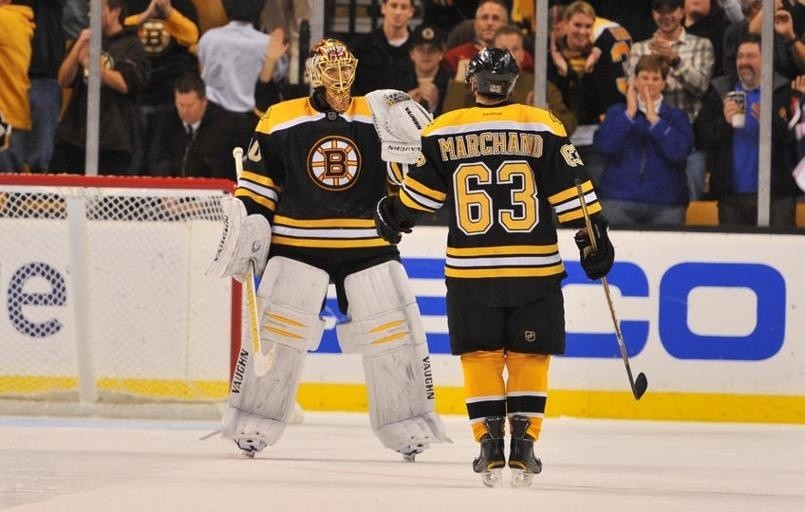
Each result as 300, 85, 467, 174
405, 23, 443, 51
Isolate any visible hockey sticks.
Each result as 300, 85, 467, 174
575, 176, 647, 399
231, 146, 277, 377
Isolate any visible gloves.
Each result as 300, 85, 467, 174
374, 195, 413, 244
573, 215, 615, 280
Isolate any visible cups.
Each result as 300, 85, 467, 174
727, 91, 747, 129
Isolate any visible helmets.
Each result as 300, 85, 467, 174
303, 37, 360, 113
463, 45, 522, 99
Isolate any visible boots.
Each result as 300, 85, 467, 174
508, 414, 543, 474
472, 415, 506, 473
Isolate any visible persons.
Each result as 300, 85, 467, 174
373, 44, 618, 475
351, 0, 579, 139
201, 35, 451, 455
2, 0, 310, 201
549, 0, 805, 232
